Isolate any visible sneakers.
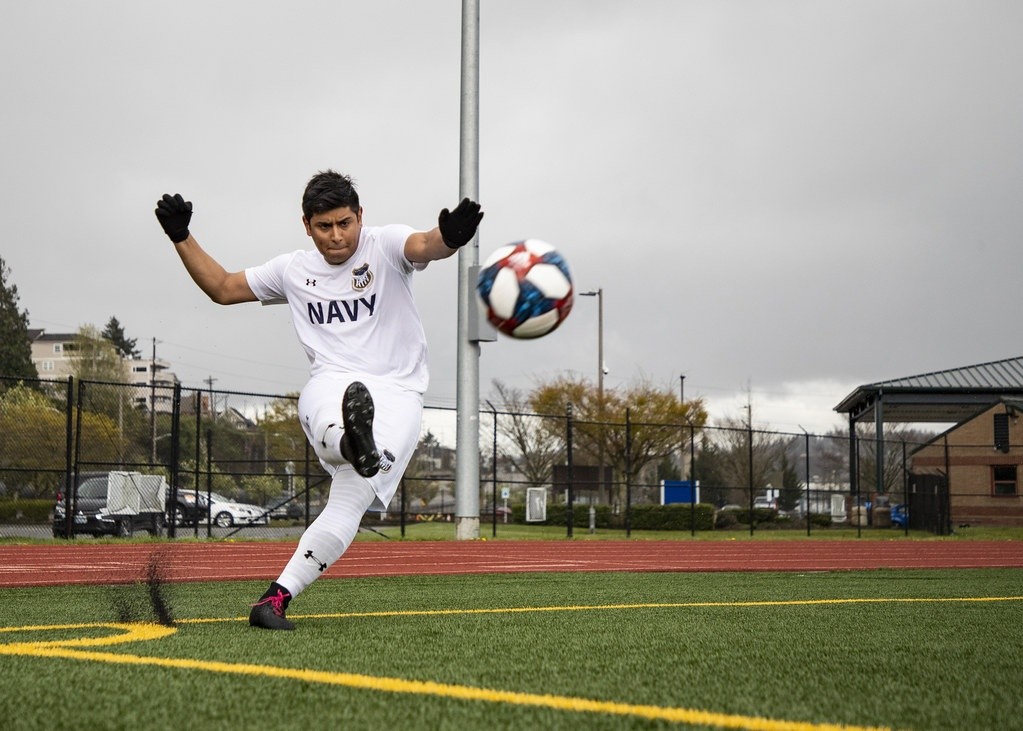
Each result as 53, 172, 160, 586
249, 583, 296, 631
342, 382, 380, 478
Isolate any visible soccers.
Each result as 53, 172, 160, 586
473, 238, 575, 342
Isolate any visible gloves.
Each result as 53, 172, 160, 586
156, 193, 195, 243
438, 197, 484, 249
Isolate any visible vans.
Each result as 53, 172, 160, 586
753, 497, 775, 508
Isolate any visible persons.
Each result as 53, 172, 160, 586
154, 172, 485, 630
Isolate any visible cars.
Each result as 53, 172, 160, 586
263, 496, 300, 521
51, 477, 163, 540
177, 489, 271, 529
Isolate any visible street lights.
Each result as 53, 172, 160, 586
579, 288, 607, 503
680, 374, 686, 455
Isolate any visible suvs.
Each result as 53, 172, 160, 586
56, 471, 208, 527
